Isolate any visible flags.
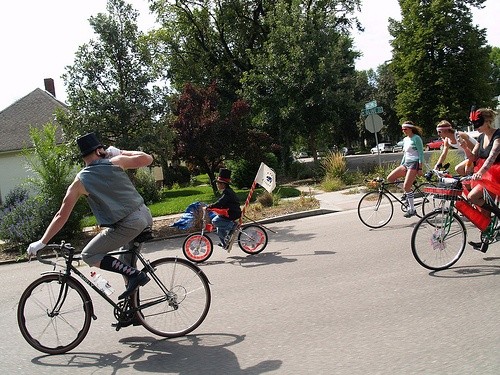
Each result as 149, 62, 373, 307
255, 162, 276, 194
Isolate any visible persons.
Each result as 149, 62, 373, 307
206, 168, 241, 249
457, 108, 500, 253
387, 121, 424, 218
433, 120, 480, 192
26, 132, 154, 327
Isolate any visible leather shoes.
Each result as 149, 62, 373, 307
112, 313, 145, 328
118, 271, 151, 299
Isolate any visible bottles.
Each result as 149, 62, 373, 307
90, 271, 115, 296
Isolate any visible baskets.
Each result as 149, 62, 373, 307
422, 181, 432, 193
433, 182, 463, 201
363, 177, 380, 189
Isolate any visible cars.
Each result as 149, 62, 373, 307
391, 143, 403, 152
370, 142, 393, 153
426, 136, 450, 150
296, 151, 308, 157
317, 150, 328, 156
339, 147, 355, 155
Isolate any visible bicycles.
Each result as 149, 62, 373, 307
358, 167, 442, 229
411, 162, 500, 270
16, 227, 211, 357
182, 207, 269, 264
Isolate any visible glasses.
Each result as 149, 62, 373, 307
402, 127, 410, 131
438, 130, 446, 133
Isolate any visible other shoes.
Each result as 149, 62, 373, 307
218, 242, 225, 247
404, 209, 417, 218
469, 241, 488, 253
228, 222, 239, 235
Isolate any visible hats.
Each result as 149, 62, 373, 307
75, 132, 106, 158
212, 168, 234, 186
